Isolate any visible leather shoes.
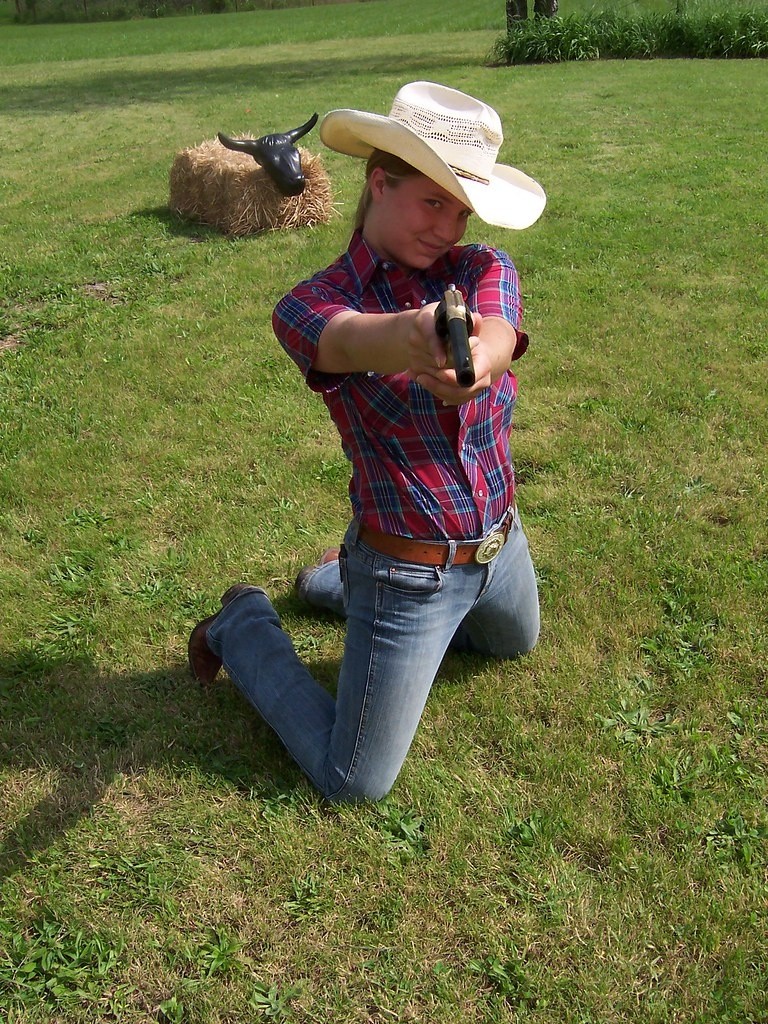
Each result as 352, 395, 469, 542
188, 583, 254, 688
294, 547, 340, 591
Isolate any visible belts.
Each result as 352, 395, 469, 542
358, 498, 516, 565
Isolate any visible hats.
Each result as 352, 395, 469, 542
320, 81, 547, 230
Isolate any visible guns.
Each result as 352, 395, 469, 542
435, 283, 475, 388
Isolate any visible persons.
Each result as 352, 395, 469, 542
186, 79, 550, 807
505, 0, 560, 32
